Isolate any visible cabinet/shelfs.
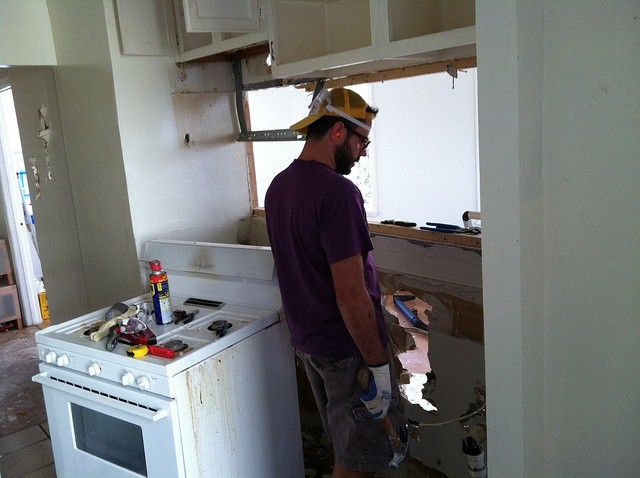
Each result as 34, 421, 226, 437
115, 0, 269, 61
269, 0, 476, 81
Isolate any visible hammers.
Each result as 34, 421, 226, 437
358, 369, 410, 469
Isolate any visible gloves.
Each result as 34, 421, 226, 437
90, 305, 137, 342
355, 361, 392, 420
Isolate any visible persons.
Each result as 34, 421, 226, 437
264, 86, 411, 477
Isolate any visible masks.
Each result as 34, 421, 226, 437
308, 88, 370, 133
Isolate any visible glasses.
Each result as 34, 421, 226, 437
344, 124, 371, 149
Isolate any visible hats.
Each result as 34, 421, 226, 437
289, 88, 377, 131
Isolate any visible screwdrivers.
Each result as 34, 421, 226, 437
117, 339, 176, 359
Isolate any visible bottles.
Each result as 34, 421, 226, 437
149, 259, 174, 326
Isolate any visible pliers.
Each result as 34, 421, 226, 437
174, 309, 199, 325
419, 222, 481, 235
380, 219, 417, 227
